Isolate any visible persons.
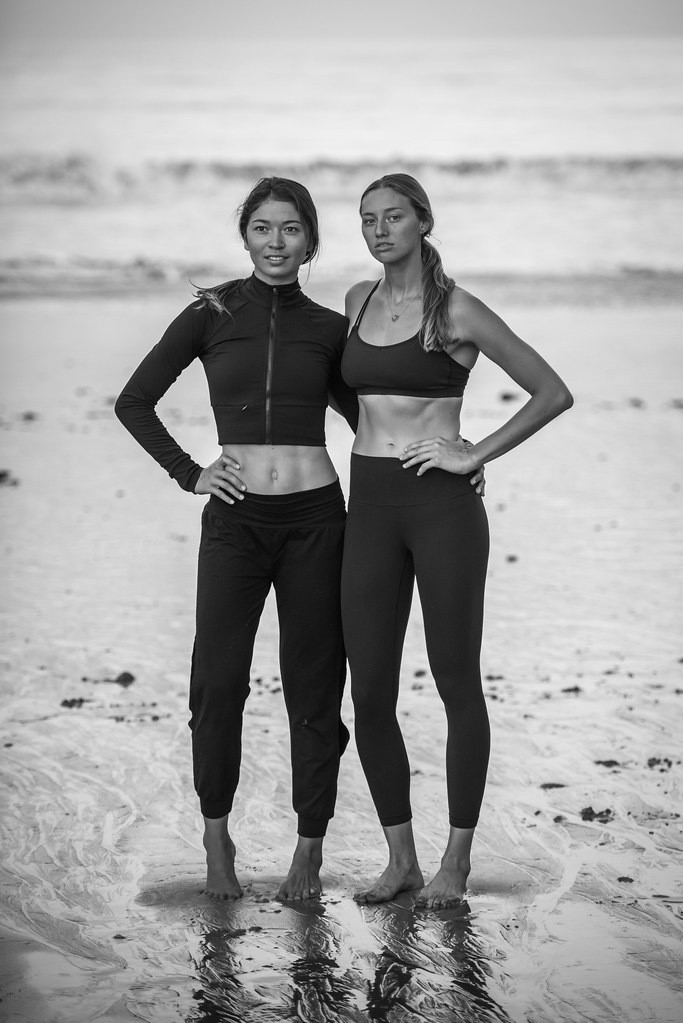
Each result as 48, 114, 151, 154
114, 173, 574, 908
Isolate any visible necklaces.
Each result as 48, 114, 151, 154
386, 286, 417, 322
